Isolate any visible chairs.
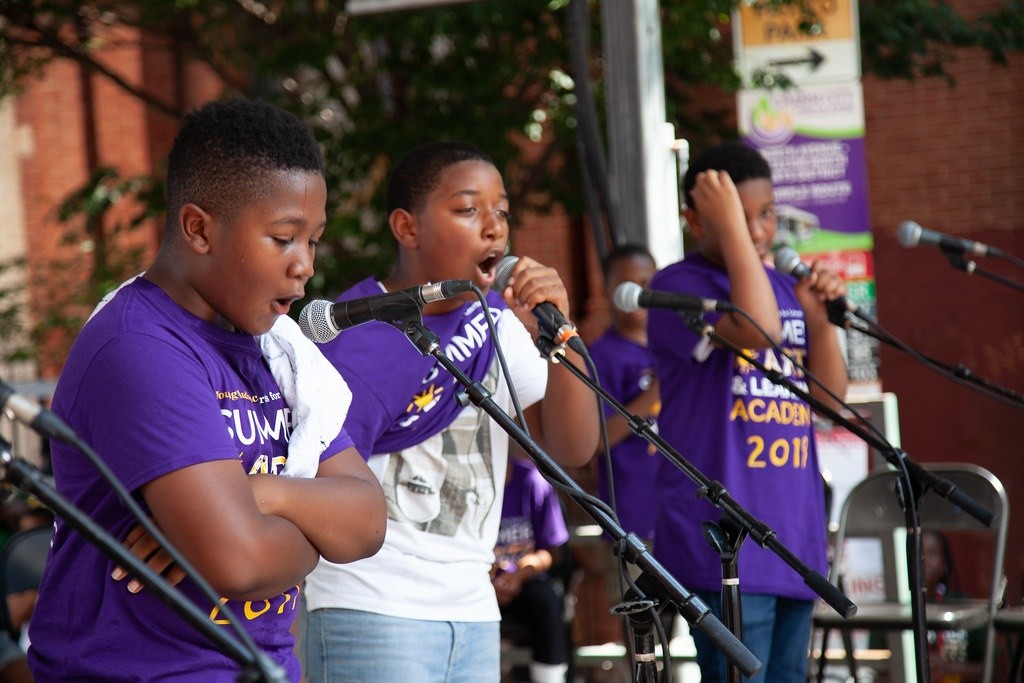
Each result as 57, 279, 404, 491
575, 461, 1024, 683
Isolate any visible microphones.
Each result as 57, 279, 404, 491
897, 219, 1006, 257
613, 281, 735, 315
772, 246, 872, 325
0, 378, 80, 446
495, 256, 588, 357
299, 278, 471, 343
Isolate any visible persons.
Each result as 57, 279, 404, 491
920, 531, 965, 602
641, 144, 851, 683
303, 138, 599, 683
486, 454, 572, 683
585, 243, 690, 683
24, 98, 388, 683
